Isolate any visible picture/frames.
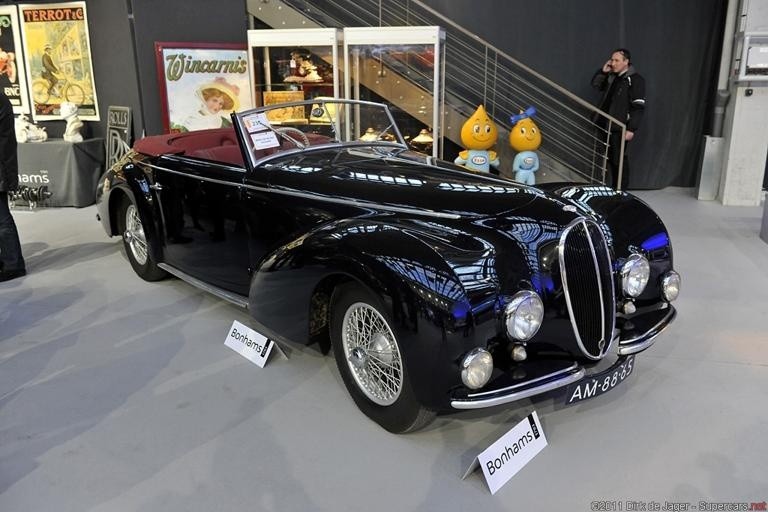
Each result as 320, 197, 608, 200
153, 41, 262, 133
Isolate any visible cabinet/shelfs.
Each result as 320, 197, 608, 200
246, 24, 447, 162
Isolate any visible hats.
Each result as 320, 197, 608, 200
198, 77, 240, 112
43, 43, 52, 50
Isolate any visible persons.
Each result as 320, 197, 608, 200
175, 78, 244, 130
0, 88, 25, 282
592, 49, 646, 192
56, 102, 86, 142
39, 43, 60, 97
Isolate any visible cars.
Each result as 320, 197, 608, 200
94, 98, 680, 434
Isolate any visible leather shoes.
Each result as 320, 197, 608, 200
0, 269, 26, 283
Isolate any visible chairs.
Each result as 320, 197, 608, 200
193, 145, 266, 168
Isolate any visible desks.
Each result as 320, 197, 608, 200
8, 136, 104, 207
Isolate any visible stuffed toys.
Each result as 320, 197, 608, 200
510, 110, 542, 185
453, 104, 501, 175
